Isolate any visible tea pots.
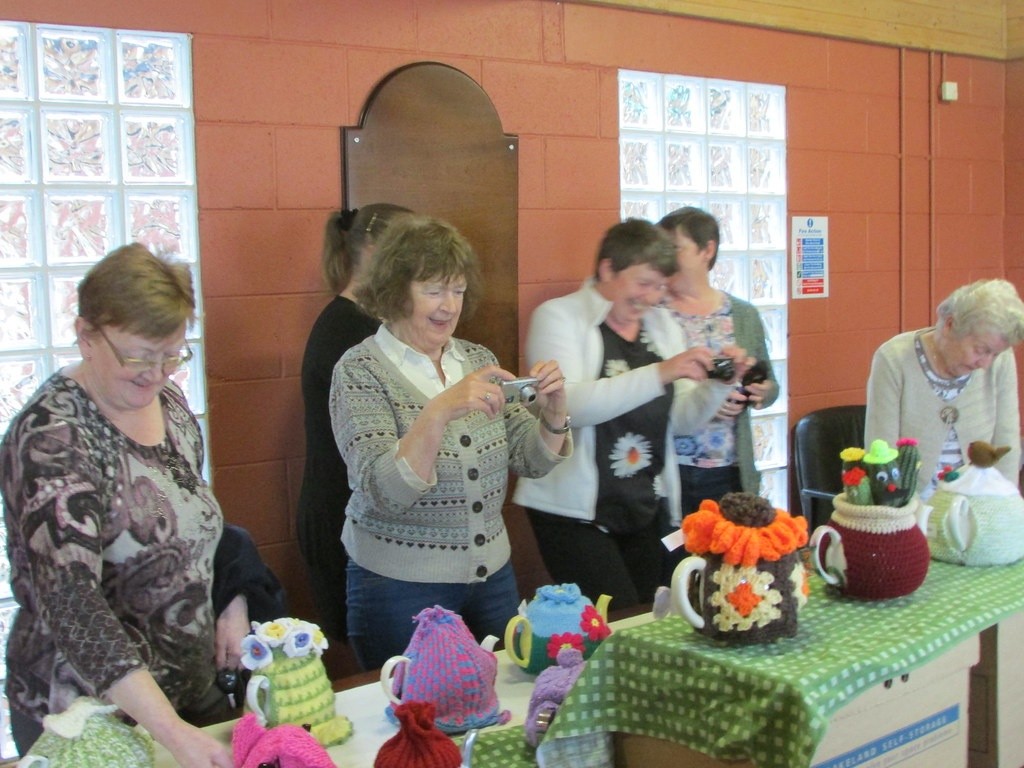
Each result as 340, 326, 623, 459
232, 714, 337, 768
525, 647, 588, 748
926, 441, 1024, 567
380, 604, 500, 733
671, 491, 810, 643
239, 616, 352, 747
374, 699, 479, 768
17, 695, 154, 768
504, 583, 613, 674
809, 436, 934, 601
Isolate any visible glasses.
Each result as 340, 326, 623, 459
79, 313, 193, 370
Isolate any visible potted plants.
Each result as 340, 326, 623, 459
809, 439, 930, 601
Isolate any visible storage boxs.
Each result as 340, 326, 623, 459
612, 602, 1024, 768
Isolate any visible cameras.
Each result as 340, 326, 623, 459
707, 358, 737, 382
499, 376, 539, 404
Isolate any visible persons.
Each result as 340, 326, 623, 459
865, 279, 1024, 501
513, 218, 747, 612
1, 242, 251, 768
657, 206, 781, 587
329, 218, 573, 668
298, 203, 415, 677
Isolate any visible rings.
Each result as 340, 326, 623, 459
484, 392, 491, 402
561, 376, 566, 382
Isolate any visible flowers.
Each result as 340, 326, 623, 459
240, 617, 328, 670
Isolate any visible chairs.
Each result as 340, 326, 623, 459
792, 404, 867, 533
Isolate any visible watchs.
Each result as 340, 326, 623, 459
540, 411, 571, 434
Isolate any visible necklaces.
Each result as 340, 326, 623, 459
931, 338, 942, 377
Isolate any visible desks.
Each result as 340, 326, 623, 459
150, 557, 670, 768
